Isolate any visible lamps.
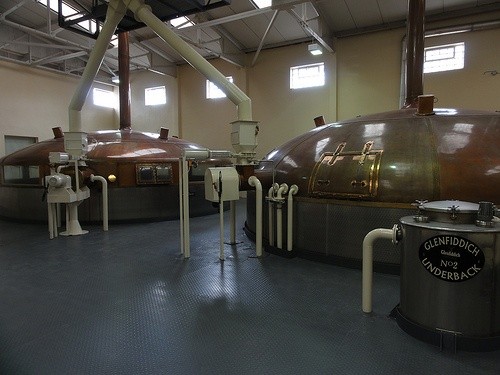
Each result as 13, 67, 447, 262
308, 44, 323, 57
